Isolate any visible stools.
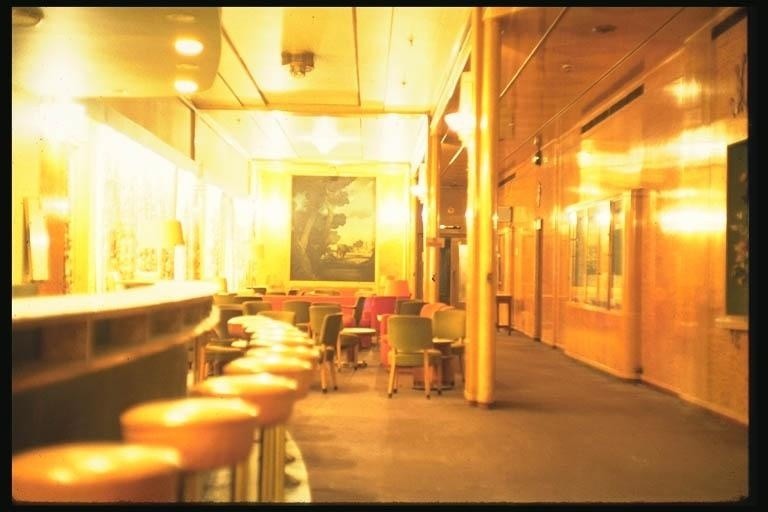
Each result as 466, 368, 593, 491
13, 372, 298, 504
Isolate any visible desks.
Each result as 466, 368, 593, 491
495, 292, 514, 335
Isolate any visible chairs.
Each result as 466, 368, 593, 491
190, 280, 468, 398
